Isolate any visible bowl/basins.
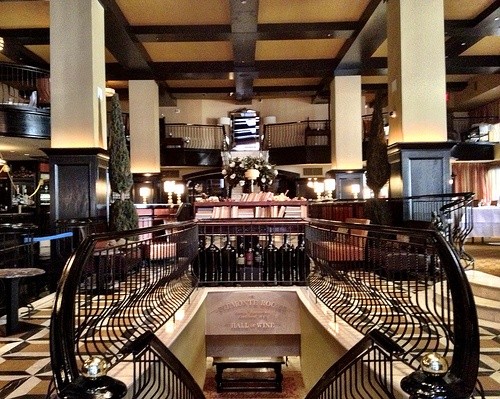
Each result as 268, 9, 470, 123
0, 108, 51, 138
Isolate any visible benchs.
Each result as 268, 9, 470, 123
313, 218, 370, 267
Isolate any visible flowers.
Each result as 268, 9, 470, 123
221, 155, 279, 191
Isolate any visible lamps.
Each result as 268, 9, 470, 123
216, 117, 232, 150
351, 184, 361, 199
314, 182, 324, 201
140, 187, 150, 204
263, 116, 276, 148
164, 181, 176, 205
106, 84, 115, 97
173, 184, 185, 204
324, 179, 336, 199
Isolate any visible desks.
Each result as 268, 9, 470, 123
463, 207, 500, 244
213, 356, 286, 392
305, 132, 330, 146
164, 137, 184, 149
0, 268, 46, 337
